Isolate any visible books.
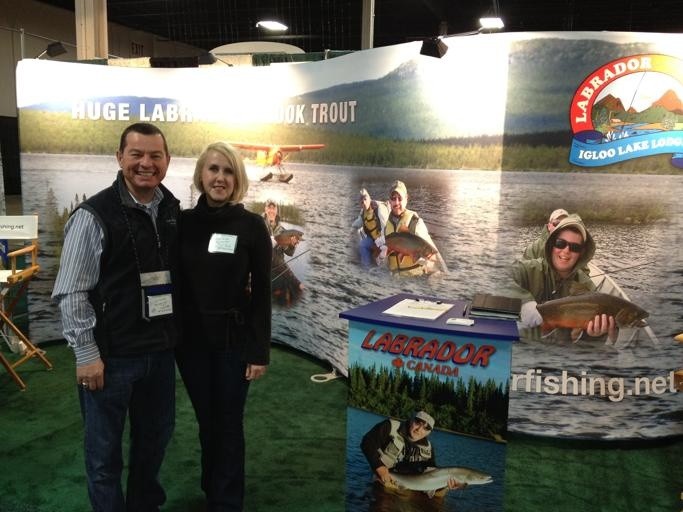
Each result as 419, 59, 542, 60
469, 294, 524, 320
384, 299, 455, 319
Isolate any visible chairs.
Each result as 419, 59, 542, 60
0, 212, 53, 391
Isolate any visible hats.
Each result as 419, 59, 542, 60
389, 180, 407, 199
560, 222, 587, 242
265, 199, 278, 208
416, 411, 434, 431
359, 188, 370, 199
550, 209, 570, 221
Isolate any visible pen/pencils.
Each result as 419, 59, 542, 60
460, 296, 471, 322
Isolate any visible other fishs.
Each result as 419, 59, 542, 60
375, 466, 494, 498
370, 231, 439, 264
275, 229, 307, 246
536, 293, 650, 333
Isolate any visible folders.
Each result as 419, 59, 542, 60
471, 293, 522, 316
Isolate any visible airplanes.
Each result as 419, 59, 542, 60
225, 137, 327, 185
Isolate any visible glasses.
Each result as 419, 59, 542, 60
554, 238, 583, 253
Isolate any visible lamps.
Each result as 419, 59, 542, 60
257, 18, 288, 33
478, 0, 505, 29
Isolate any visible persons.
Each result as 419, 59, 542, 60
348, 186, 394, 273
50, 124, 172, 512
386, 180, 449, 285
525, 208, 569, 259
175, 141, 274, 512
360, 410, 465, 497
260, 198, 302, 306
268, 147, 291, 176
500, 213, 649, 355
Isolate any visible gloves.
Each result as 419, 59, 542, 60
374, 236, 388, 257
520, 302, 543, 328
